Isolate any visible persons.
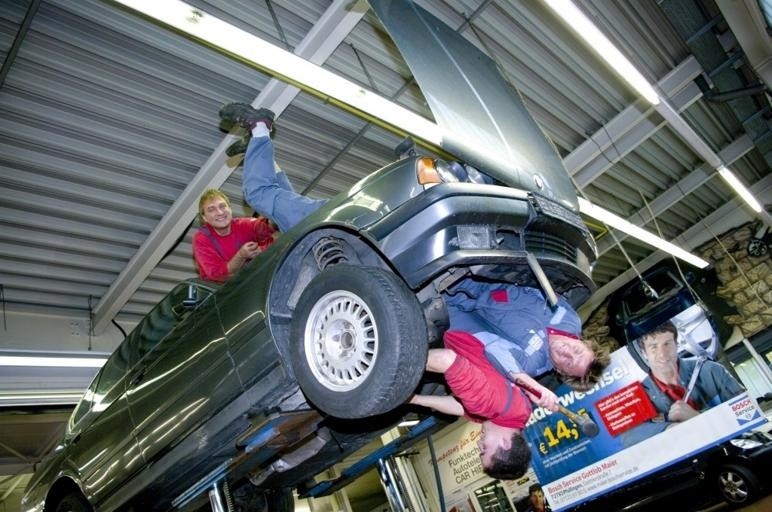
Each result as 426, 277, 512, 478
408, 332, 533, 481
619, 319, 745, 450
191, 188, 275, 282
528, 484, 553, 512
218, 101, 333, 234
441, 279, 613, 412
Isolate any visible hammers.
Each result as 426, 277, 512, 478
514, 379, 598, 437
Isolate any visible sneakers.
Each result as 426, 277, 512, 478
219, 103, 275, 132
226, 127, 277, 157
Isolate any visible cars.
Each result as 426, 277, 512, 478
570, 409, 772, 512
614, 264, 732, 345
21, 154, 599, 512
